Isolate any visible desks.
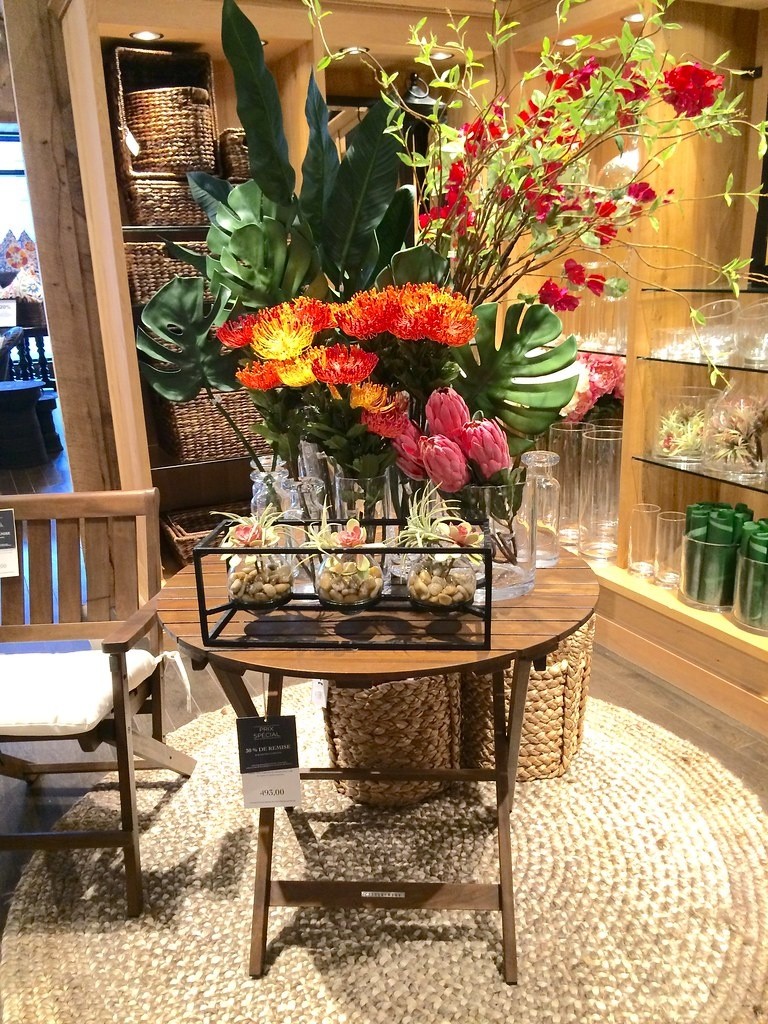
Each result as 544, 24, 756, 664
0, 381, 50, 471
154, 523, 600, 985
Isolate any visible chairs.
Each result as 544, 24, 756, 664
1, 488, 198, 920
0, 326, 24, 382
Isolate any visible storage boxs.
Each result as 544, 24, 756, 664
16, 299, 48, 327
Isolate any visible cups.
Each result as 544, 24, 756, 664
677, 530, 768, 638
653, 511, 687, 589
650, 296, 768, 370
626, 501, 661, 578
548, 418, 623, 557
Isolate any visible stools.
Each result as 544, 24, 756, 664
36, 394, 64, 454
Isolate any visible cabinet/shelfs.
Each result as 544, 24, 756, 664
506, 0, 768, 662
42, 0, 514, 488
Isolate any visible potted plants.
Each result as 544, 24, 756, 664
380, 478, 485, 607
286, 492, 386, 606
209, 502, 313, 606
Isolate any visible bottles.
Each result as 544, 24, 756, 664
555, 260, 627, 354
519, 449, 563, 569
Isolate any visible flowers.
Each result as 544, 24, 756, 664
134, 0, 768, 577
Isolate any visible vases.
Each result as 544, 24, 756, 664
223, 263, 767, 636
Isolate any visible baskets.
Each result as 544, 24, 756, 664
164, 386, 276, 465
146, 326, 233, 372
323, 608, 593, 805
125, 241, 248, 310
111, 46, 216, 179
219, 128, 250, 181
123, 179, 211, 226
161, 500, 251, 567
